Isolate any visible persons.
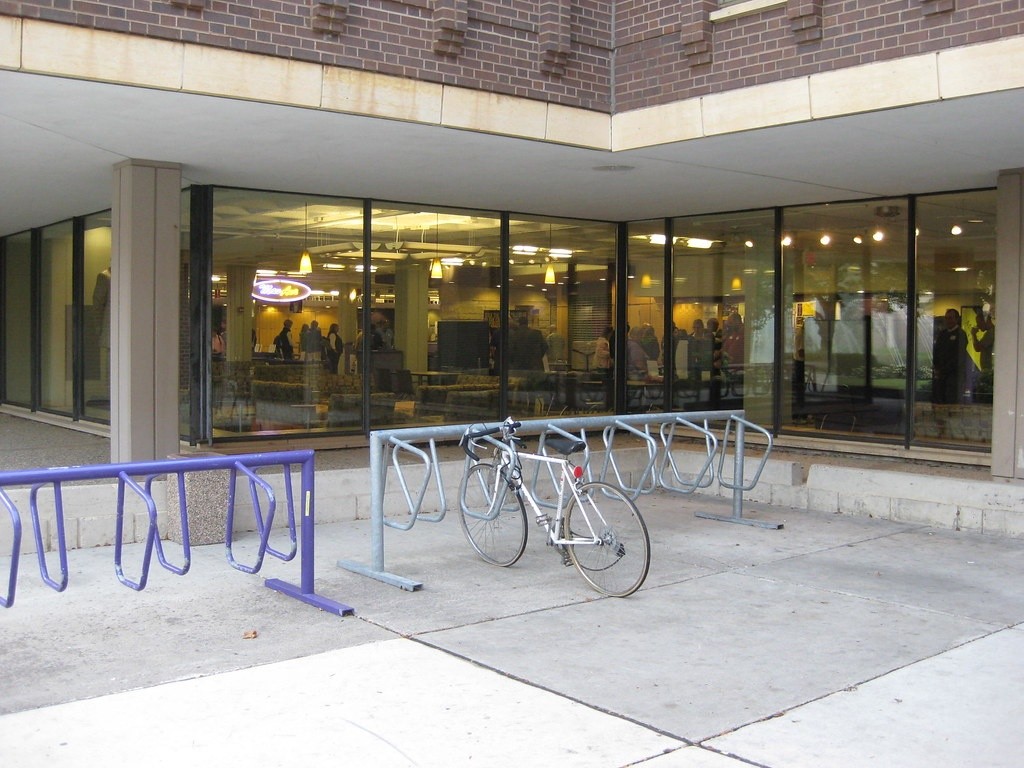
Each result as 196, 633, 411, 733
251, 325, 257, 353
273, 319, 394, 375
491, 316, 565, 417
592, 313, 744, 382
791, 317, 817, 424
211, 319, 228, 353
92, 258, 111, 401
933, 309, 994, 403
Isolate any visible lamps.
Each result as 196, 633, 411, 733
299, 202, 312, 275
730, 275, 742, 290
544, 223, 556, 284
430, 211, 442, 279
641, 247, 652, 289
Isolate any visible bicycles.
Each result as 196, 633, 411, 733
456, 417, 651, 599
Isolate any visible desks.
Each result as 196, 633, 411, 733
718, 364, 794, 398
409, 370, 463, 387
582, 378, 665, 412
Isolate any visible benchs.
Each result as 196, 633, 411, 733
209, 361, 701, 440
